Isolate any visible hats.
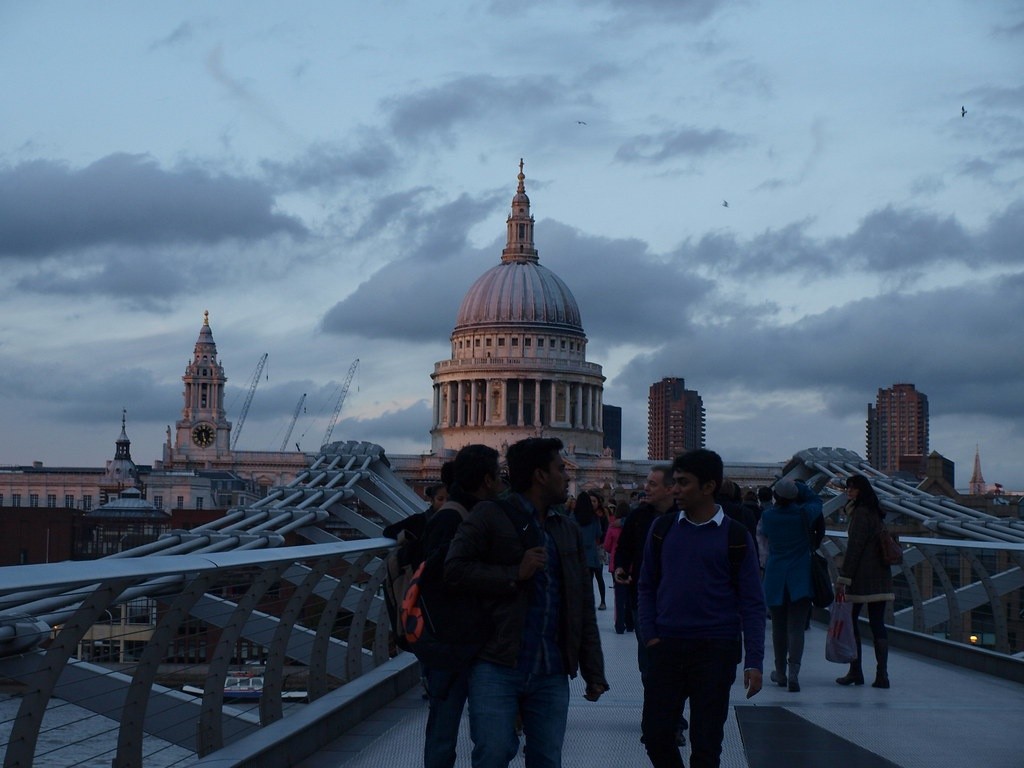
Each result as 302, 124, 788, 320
775, 479, 799, 498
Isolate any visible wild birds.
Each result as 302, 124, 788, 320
576, 120, 587, 125
721, 199, 728, 207
961, 105, 967, 117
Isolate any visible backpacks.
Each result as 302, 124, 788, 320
383, 500, 469, 654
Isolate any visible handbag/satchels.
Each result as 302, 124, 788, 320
811, 552, 834, 608
400, 497, 537, 671
826, 592, 858, 663
880, 528, 903, 565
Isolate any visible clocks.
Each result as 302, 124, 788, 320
192, 424, 216, 449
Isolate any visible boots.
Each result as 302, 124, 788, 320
788, 663, 800, 692
872, 639, 889, 688
770, 655, 787, 687
836, 639, 864, 685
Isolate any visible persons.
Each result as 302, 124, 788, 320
756, 479, 824, 691
637, 450, 766, 768
419, 444, 503, 768
835, 474, 896, 688
442, 436, 609, 768
386, 462, 772, 734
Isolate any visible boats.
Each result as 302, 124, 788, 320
182, 672, 307, 705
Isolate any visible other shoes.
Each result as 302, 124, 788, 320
627, 628, 633, 632
675, 729, 687, 746
616, 627, 624, 634
598, 602, 606, 610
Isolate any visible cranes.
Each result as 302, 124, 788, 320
280, 393, 307, 452
230, 352, 268, 452
321, 358, 361, 447
